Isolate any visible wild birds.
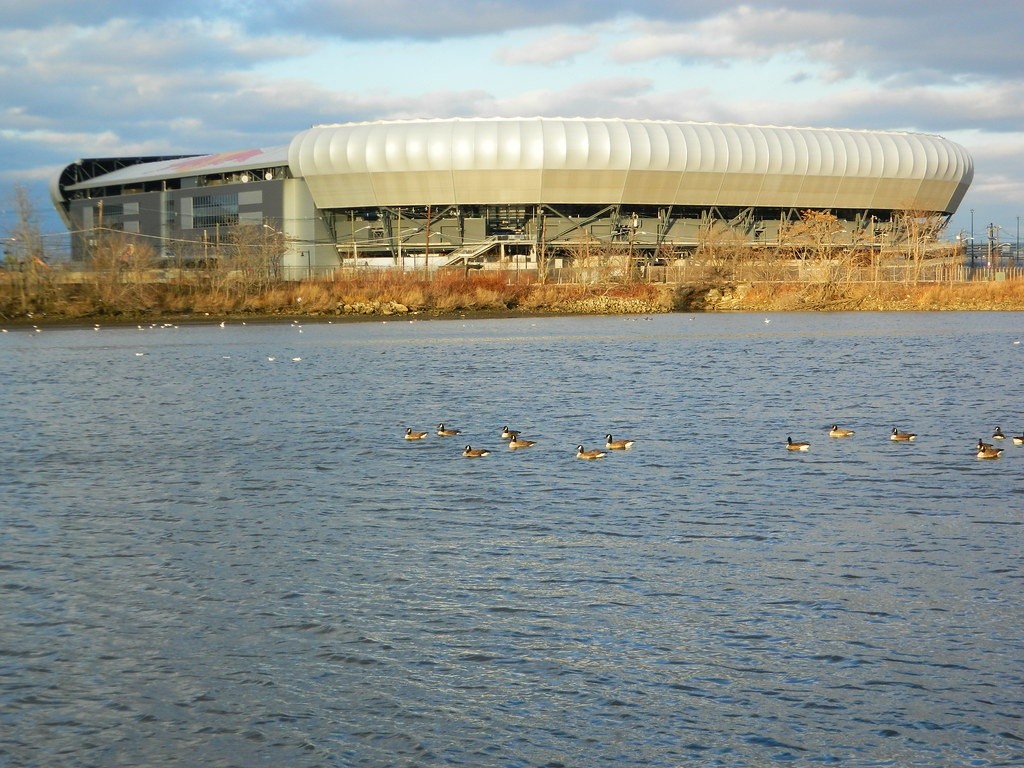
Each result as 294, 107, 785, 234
785, 423, 920, 453
403, 421, 539, 460
976, 424, 1024, 461
576, 432, 636, 461
